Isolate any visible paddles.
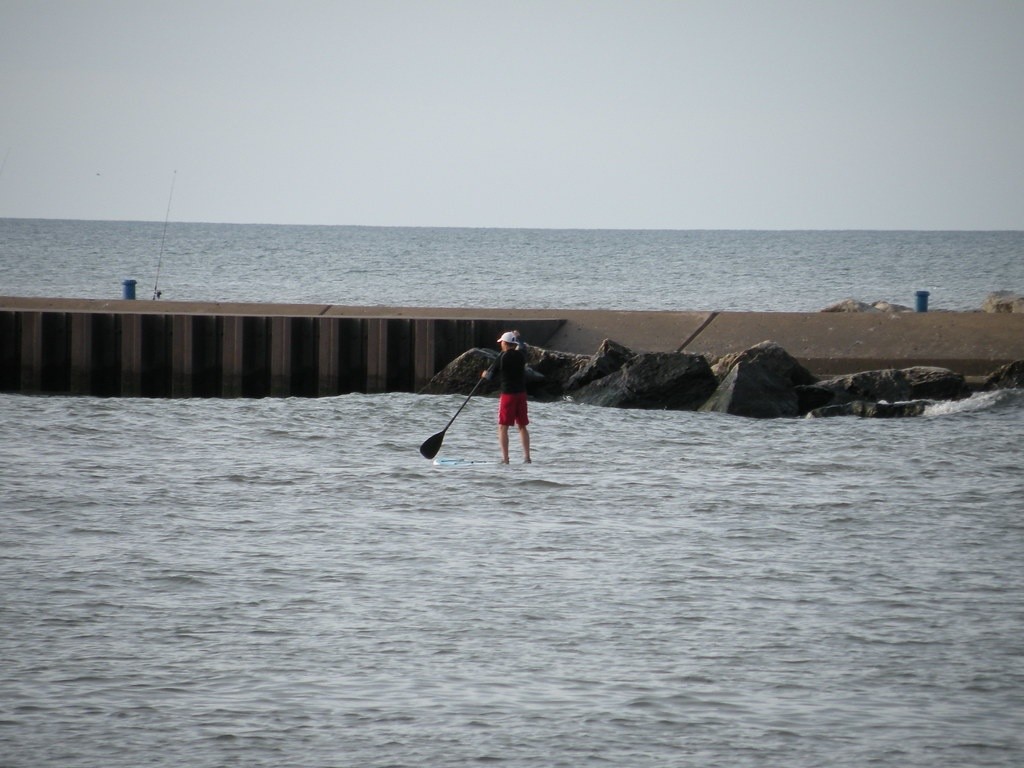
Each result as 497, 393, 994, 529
420, 361, 496, 460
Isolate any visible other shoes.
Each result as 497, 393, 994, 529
523, 460, 531, 464
500, 458, 509, 464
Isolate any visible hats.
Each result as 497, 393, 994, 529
497, 332, 519, 345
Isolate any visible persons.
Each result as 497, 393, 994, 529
482, 330, 531, 465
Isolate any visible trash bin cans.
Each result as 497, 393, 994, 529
915, 291, 930, 312
122, 279, 137, 300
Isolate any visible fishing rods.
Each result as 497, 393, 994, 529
151, 168, 177, 301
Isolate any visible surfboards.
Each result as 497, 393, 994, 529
433, 459, 493, 465
